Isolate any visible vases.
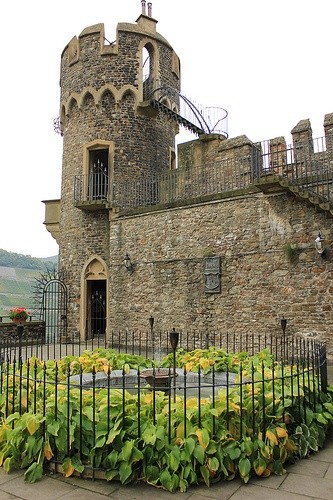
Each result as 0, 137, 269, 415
11, 317, 27, 323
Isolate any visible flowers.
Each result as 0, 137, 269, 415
9, 306, 31, 318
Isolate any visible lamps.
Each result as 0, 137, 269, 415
314, 231, 326, 254
123, 252, 132, 269
